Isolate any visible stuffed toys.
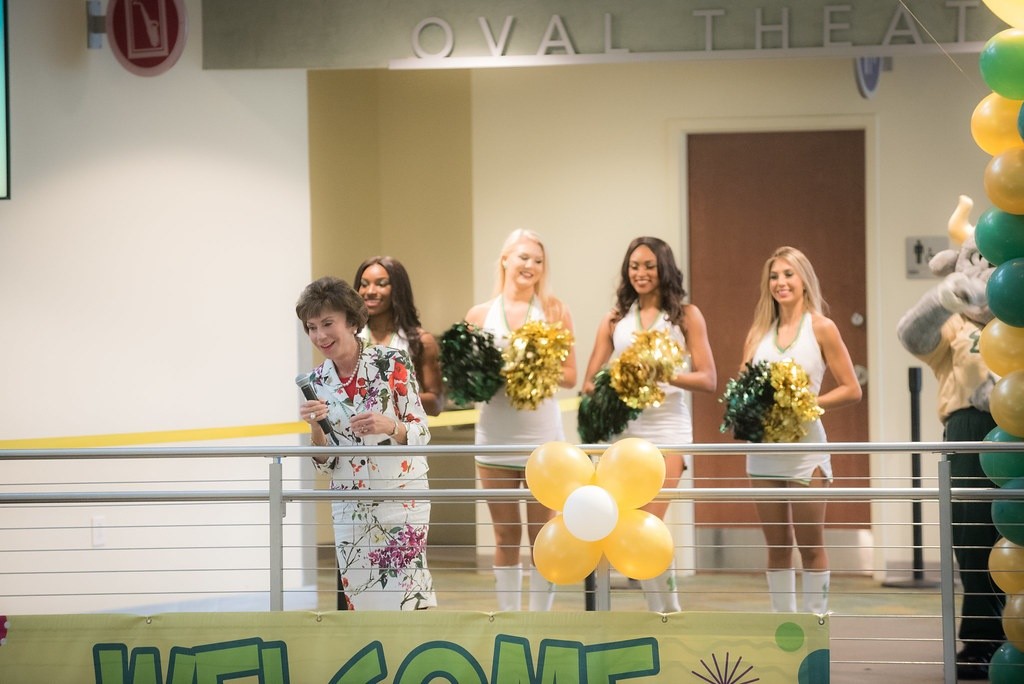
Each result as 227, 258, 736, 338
897, 194, 1011, 684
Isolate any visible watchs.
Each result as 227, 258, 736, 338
387, 418, 399, 437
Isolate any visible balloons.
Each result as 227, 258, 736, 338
533, 514, 603, 586
595, 438, 667, 510
602, 510, 674, 580
526, 440, 596, 513
972, 0, 1024, 684
563, 486, 619, 542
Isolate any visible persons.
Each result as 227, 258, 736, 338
737, 245, 861, 615
580, 237, 716, 613
466, 229, 578, 611
296, 277, 438, 611
338, 255, 444, 611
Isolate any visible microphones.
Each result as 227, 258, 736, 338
295, 373, 333, 436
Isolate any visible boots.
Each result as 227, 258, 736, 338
802, 571, 829, 616
654, 563, 679, 612
765, 569, 797, 612
527, 564, 556, 613
640, 578, 660, 613
492, 564, 523, 612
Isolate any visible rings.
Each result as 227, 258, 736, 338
310, 412, 315, 419
360, 425, 367, 432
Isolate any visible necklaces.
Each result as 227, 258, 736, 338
341, 340, 363, 387
